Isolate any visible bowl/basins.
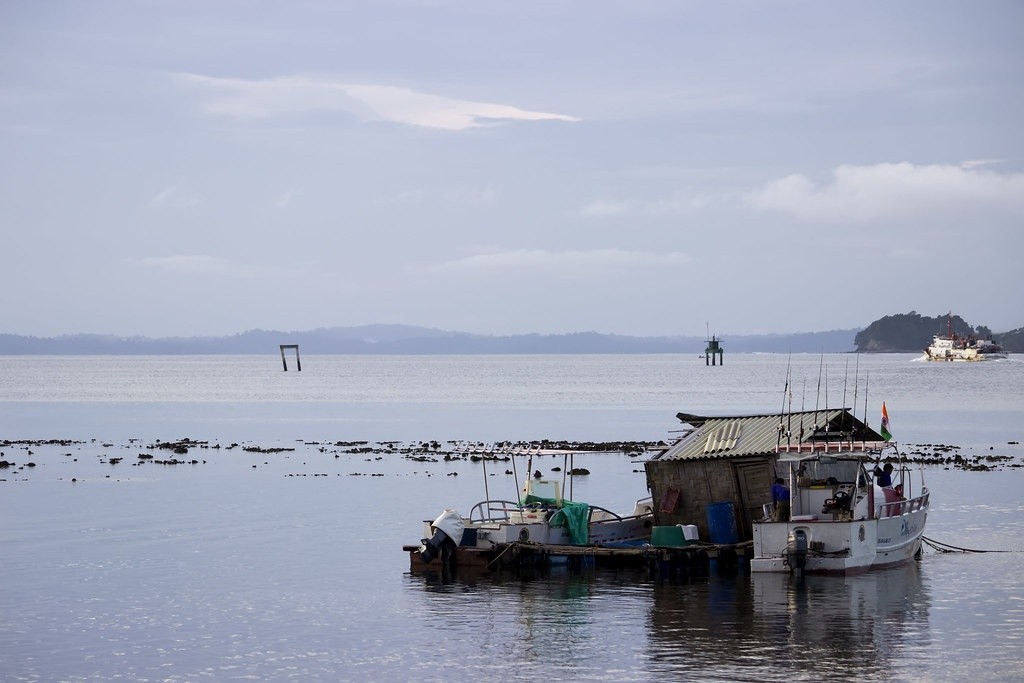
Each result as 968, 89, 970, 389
650, 525, 698, 547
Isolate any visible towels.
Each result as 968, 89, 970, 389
676, 524, 699, 540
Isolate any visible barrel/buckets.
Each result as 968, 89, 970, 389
704, 500, 738, 544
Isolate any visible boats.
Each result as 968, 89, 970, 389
421, 446, 655, 552
923, 312, 1006, 362
750, 449, 930, 575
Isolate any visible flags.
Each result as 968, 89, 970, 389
880, 403, 892, 441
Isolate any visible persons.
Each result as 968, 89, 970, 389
876, 457, 893, 489
772, 478, 790, 522
